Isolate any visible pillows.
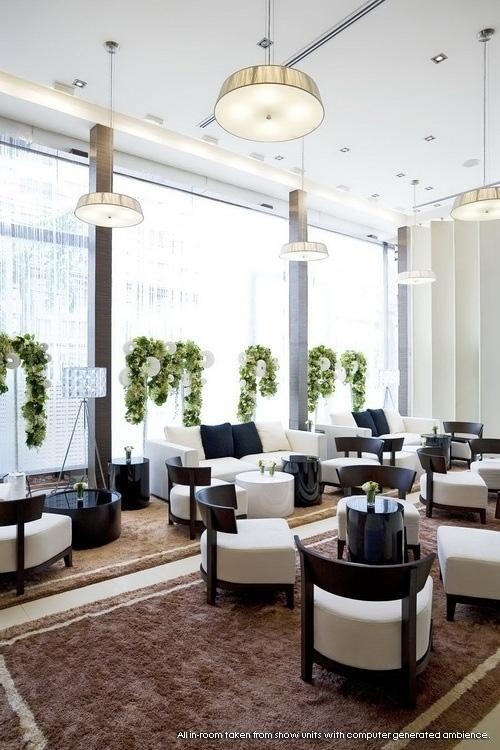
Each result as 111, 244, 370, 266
367, 408, 390, 435
351, 411, 379, 437
383, 408, 406, 433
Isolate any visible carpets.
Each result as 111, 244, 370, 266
1, 497, 499, 750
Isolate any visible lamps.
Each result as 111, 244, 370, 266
397, 179, 437, 285
450, 29, 500, 221
54, 366, 108, 490
75, 40, 144, 228
214, 0, 325, 144
278, 137, 329, 261
